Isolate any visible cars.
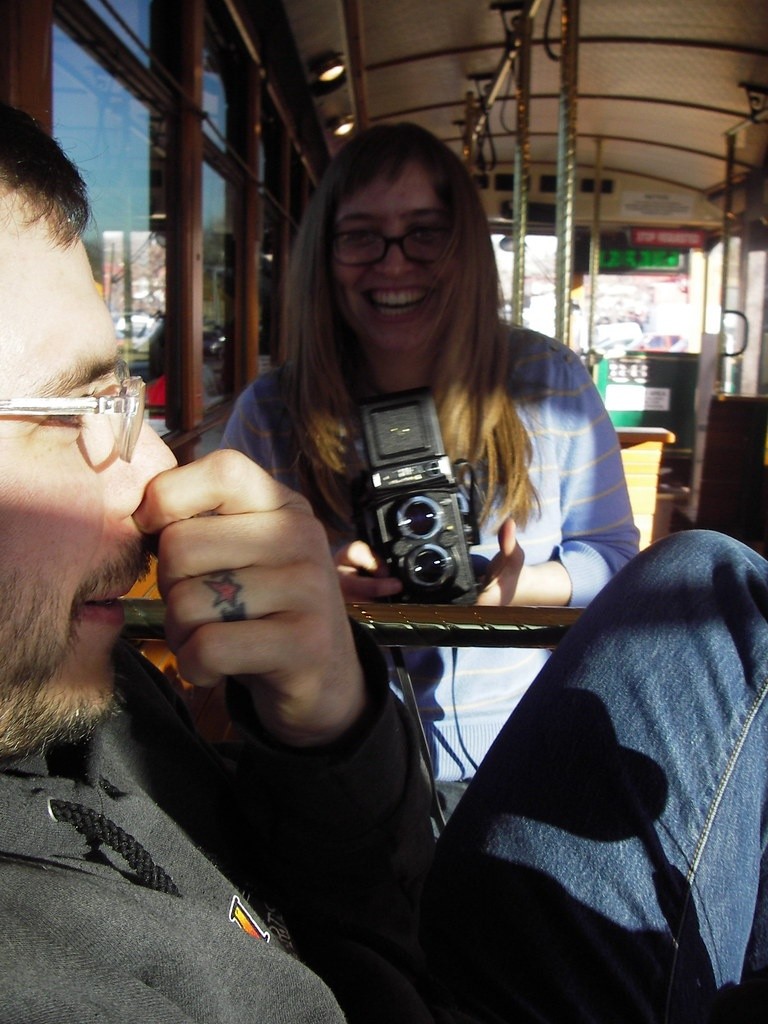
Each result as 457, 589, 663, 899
111, 315, 165, 374
576, 321, 694, 368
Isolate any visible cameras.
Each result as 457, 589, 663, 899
352, 385, 479, 607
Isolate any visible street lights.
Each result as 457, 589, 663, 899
119, 211, 166, 389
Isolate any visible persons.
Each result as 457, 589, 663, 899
227, 122, 638, 781
0, 99, 768, 1024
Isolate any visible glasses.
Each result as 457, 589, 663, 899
327, 222, 453, 265
1, 375, 145, 473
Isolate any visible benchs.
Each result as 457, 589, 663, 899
615, 392, 768, 550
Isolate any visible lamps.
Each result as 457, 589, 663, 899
308, 51, 349, 85
323, 106, 357, 138
